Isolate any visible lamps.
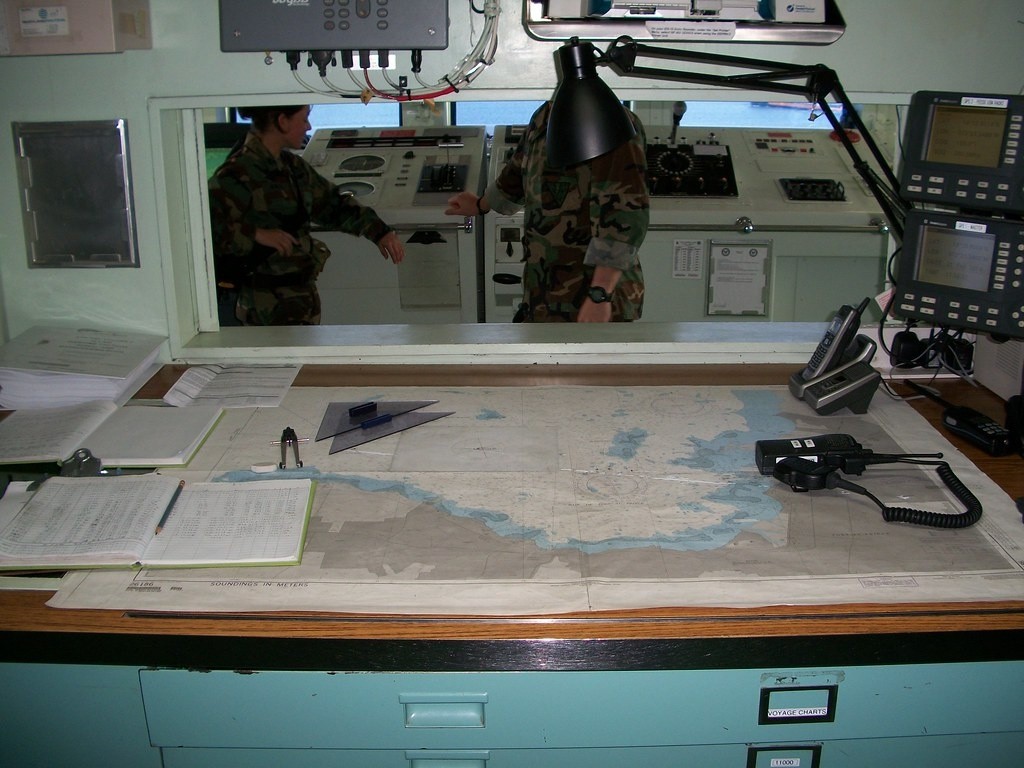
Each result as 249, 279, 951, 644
544, 32, 915, 243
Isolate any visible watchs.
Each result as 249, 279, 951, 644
586, 285, 618, 304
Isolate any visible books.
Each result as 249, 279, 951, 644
0, 476, 318, 570
0, 400, 228, 468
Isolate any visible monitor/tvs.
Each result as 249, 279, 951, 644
915, 226, 996, 292
926, 104, 1007, 168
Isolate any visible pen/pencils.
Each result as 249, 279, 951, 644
154, 480, 185, 536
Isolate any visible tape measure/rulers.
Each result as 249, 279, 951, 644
314, 400, 440, 442
328, 411, 456, 455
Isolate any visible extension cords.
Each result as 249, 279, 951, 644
853, 321, 976, 370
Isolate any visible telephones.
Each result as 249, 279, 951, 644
788, 295, 882, 416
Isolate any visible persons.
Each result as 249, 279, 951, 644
206, 104, 406, 326
443, 101, 651, 323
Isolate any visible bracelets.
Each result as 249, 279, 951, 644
476, 197, 490, 216
